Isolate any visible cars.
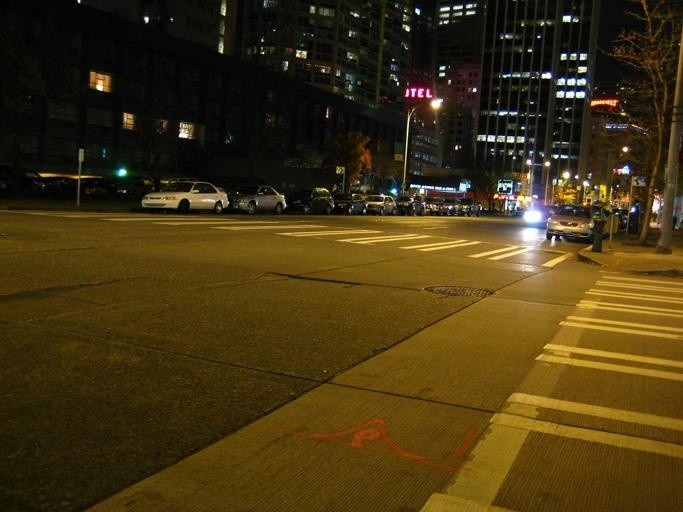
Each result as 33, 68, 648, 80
520, 201, 626, 241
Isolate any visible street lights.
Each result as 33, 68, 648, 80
526, 159, 532, 214
401, 98, 441, 195
544, 161, 552, 205
